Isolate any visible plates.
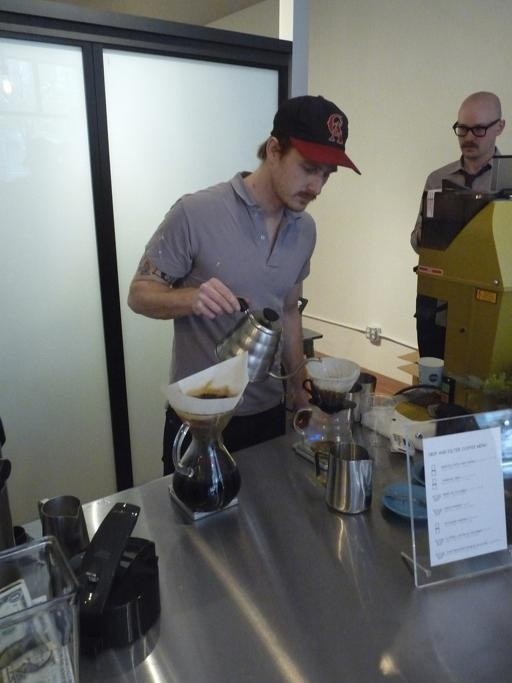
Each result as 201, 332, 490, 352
381, 482, 429, 521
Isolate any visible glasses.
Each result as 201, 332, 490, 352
452, 120, 498, 139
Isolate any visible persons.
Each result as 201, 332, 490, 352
407, 90, 512, 362
123, 92, 364, 477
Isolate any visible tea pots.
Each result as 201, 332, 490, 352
211, 296, 321, 385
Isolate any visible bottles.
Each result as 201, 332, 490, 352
170, 401, 242, 513
348, 373, 378, 423
291, 378, 358, 466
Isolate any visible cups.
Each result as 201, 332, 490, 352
360, 392, 400, 449
314, 442, 375, 516
417, 356, 445, 388
36, 494, 90, 554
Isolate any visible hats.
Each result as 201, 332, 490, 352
272, 96, 363, 176
